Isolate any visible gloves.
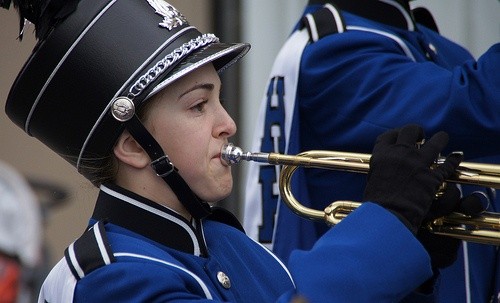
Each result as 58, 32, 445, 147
364, 125, 464, 236
419, 189, 482, 269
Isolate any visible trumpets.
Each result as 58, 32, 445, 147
220, 143, 500, 243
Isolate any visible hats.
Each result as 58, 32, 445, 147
0, 0, 252, 187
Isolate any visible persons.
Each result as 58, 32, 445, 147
242, 1, 500, 302
0, 156, 68, 303
5, 0, 484, 303
